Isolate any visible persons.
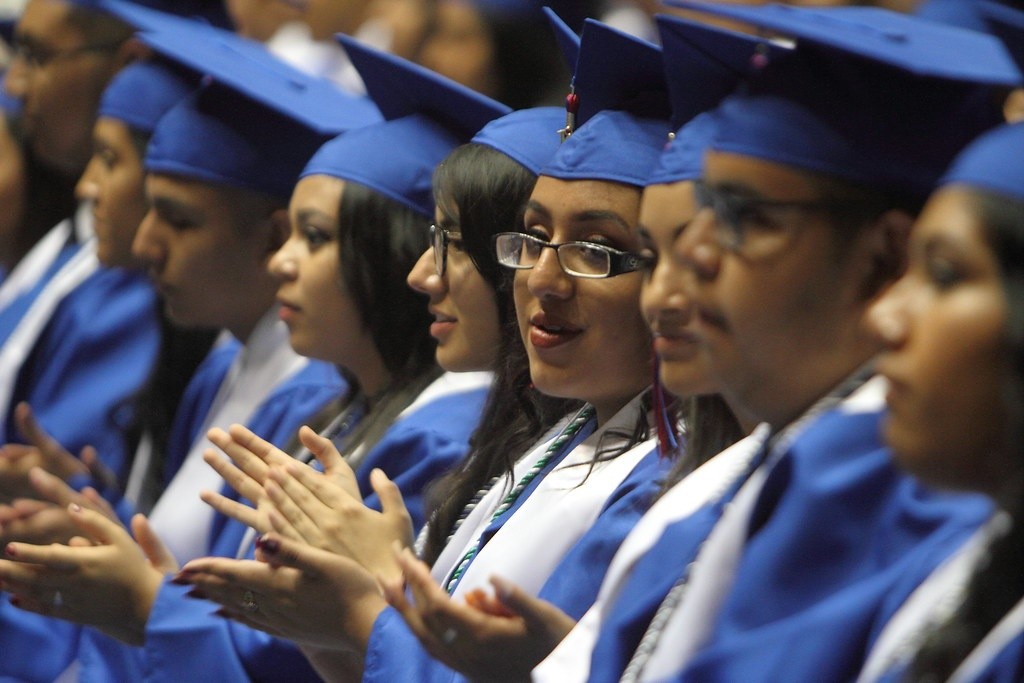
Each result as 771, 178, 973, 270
0, 0, 1024, 683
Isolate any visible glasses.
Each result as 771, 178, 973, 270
433, 226, 462, 276
493, 232, 638, 278
9, 38, 121, 68
694, 181, 830, 262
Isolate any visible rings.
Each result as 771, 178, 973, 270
242, 602, 264, 623
441, 628, 459, 650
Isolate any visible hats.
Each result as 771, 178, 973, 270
665, 0, 1024, 189
471, 5, 581, 177
299, 33, 514, 216
145, 30, 387, 200
923, 0, 1024, 34
539, 18, 663, 187
938, 120, 1024, 200
96, 15, 210, 132
647, 13, 794, 183
0, 18, 25, 111
61, 0, 180, 31
